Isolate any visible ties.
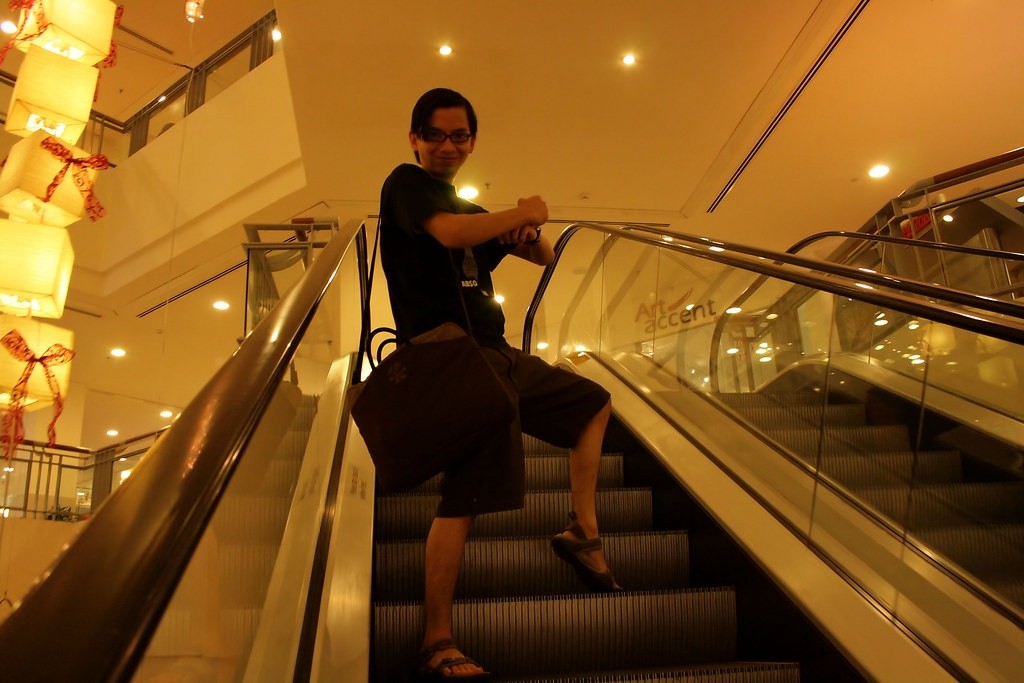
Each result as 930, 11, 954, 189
447, 185, 478, 282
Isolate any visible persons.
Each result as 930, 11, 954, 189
380, 88, 622, 683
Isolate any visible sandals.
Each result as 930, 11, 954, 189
551, 511, 623, 593
415, 639, 490, 681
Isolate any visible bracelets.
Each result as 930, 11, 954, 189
524, 227, 541, 246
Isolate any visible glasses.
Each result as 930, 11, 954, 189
419, 128, 471, 143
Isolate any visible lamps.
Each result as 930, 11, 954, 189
185, 0, 203, 23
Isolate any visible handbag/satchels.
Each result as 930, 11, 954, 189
346, 323, 519, 495
